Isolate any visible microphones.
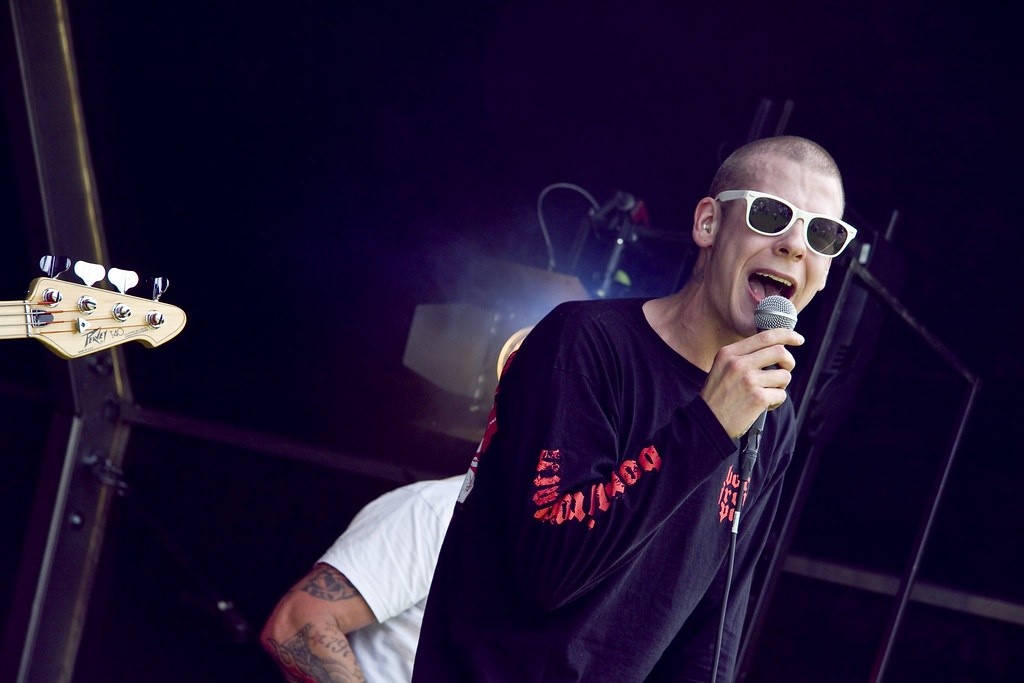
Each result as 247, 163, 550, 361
730, 295, 797, 533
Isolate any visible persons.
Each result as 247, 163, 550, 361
259, 325, 533, 682
409, 135, 845, 683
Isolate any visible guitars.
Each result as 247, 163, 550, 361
0, 252, 188, 362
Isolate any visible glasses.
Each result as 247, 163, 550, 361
715, 189, 858, 259
